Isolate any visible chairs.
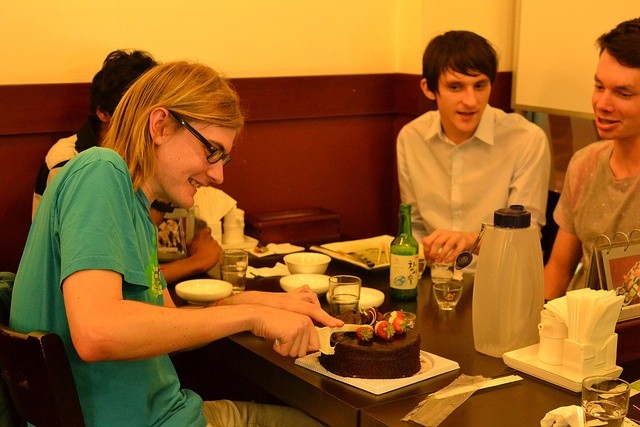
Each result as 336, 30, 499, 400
2, 328, 85, 426
391, 115, 415, 236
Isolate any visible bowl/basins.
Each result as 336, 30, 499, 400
283, 251, 332, 275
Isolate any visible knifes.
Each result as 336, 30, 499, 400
275, 323, 373, 355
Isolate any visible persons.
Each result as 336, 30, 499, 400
543, 16, 640, 303
7, 59, 346, 426
158, 218, 173, 246
396, 29, 551, 264
166, 219, 181, 247
29, 48, 225, 291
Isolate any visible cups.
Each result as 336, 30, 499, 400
430, 264, 453, 285
219, 248, 248, 290
582, 375, 630, 427
433, 282, 464, 311
329, 274, 362, 316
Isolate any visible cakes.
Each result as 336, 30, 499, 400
317, 305, 421, 377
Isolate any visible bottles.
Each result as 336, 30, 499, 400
455, 204, 544, 359
389, 203, 420, 300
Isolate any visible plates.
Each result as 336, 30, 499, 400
175, 278, 234, 305
326, 286, 386, 310
503, 342, 624, 393
309, 234, 424, 271
280, 273, 331, 294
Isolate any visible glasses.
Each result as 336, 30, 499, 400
169, 111, 232, 166
